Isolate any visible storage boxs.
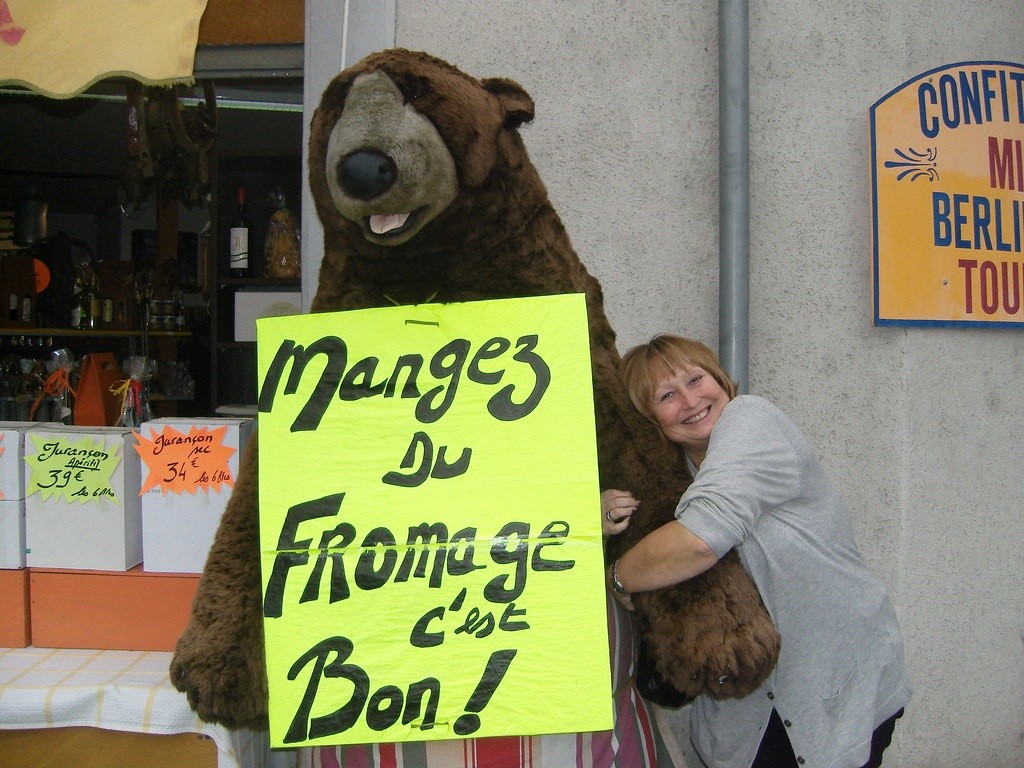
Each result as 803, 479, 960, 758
24, 424, 140, 572
142, 416, 250, 573
0, 415, 64, 570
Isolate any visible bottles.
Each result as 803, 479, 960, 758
227, 188, 301, 282
0, 255, 204, 425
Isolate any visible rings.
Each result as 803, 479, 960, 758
606, 512, 611, 519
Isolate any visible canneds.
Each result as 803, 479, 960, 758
147, 299, 180, 332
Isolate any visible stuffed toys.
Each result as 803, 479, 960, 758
169, 50, 782, 768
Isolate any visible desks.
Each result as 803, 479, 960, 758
1, 644, 272, 768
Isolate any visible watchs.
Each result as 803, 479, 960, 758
611, 559, 627, 594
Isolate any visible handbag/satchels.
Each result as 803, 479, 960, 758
73, 352, 123, 427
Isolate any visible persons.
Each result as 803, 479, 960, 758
594, 334, 915, 768
